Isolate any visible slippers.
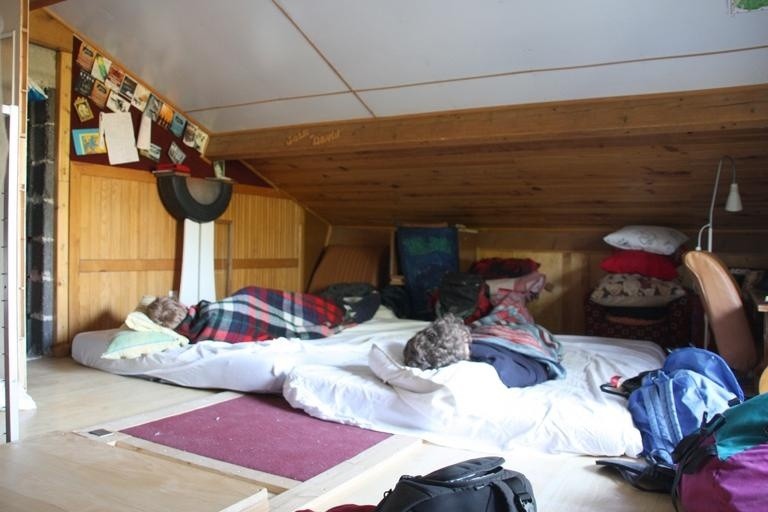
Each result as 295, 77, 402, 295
596, 460, 674, 493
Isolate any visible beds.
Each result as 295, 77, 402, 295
69, 318, 432, 394
282, 304, 667, 458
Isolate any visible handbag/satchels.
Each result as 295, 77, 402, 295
675, 392, 768, 512
373, 457, 535, 511
431, 272, 493, 324
324, 283, 381, 324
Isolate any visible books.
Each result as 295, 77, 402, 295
151, 163, 192, 177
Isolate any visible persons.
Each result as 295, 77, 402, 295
146, 284, 381, 344
404, 289, 566, 386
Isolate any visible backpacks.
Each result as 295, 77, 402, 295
631, 348, 744, 466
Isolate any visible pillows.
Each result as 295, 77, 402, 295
590, 225, 690, 326
125, 297, 190, 346
368, 343, 508, 392
470, 258, 541, 279
100, 323, 180, 360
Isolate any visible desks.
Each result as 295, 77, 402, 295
0, 431, 269, 511
746, 288, 768, 368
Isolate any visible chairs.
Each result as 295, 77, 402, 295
682, 250, 764, 398
307, 246, 384, 294
584, 287, 712, 349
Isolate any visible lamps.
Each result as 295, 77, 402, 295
695, 155, 743, 350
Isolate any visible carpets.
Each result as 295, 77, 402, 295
119, 394, 393, 482
